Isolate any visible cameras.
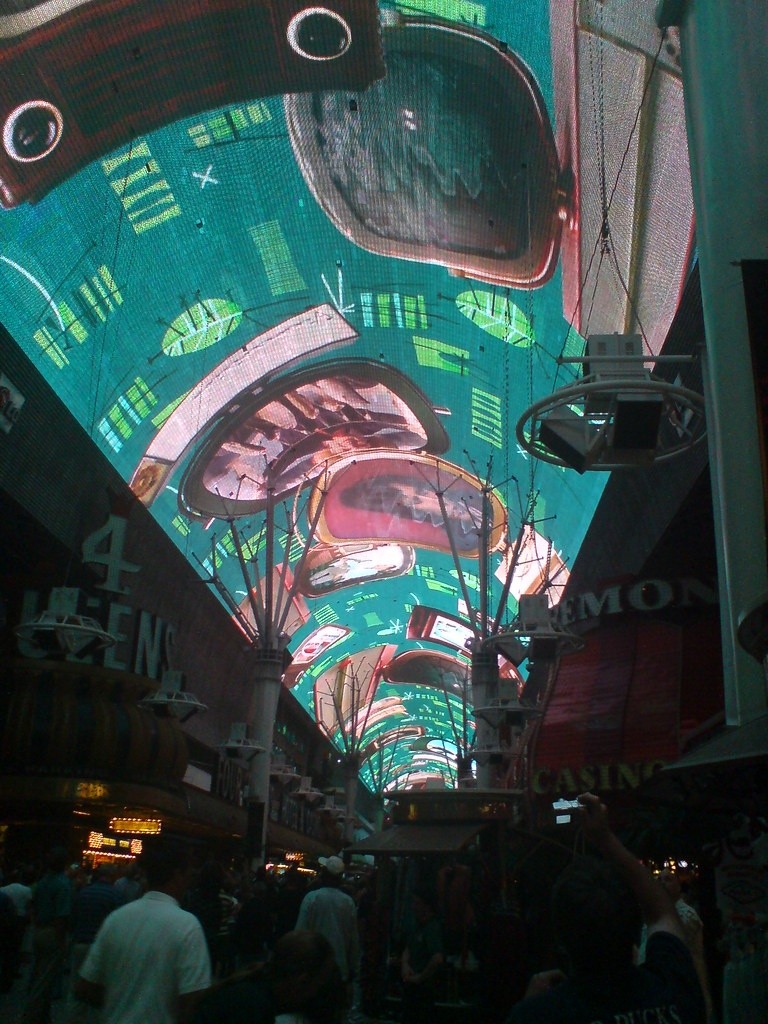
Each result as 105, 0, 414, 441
551, 799, 586, 826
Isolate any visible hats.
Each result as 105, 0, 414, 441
318, 856, 345, 875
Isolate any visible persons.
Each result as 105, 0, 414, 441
0, 790, 768, 1024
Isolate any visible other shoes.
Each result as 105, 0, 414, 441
52, 988, 63, 1001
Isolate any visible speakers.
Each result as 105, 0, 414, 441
609, 393, 664, 450
582, 334, 644, 401
470, 593, 567, 768
538, 405, 607, 475
